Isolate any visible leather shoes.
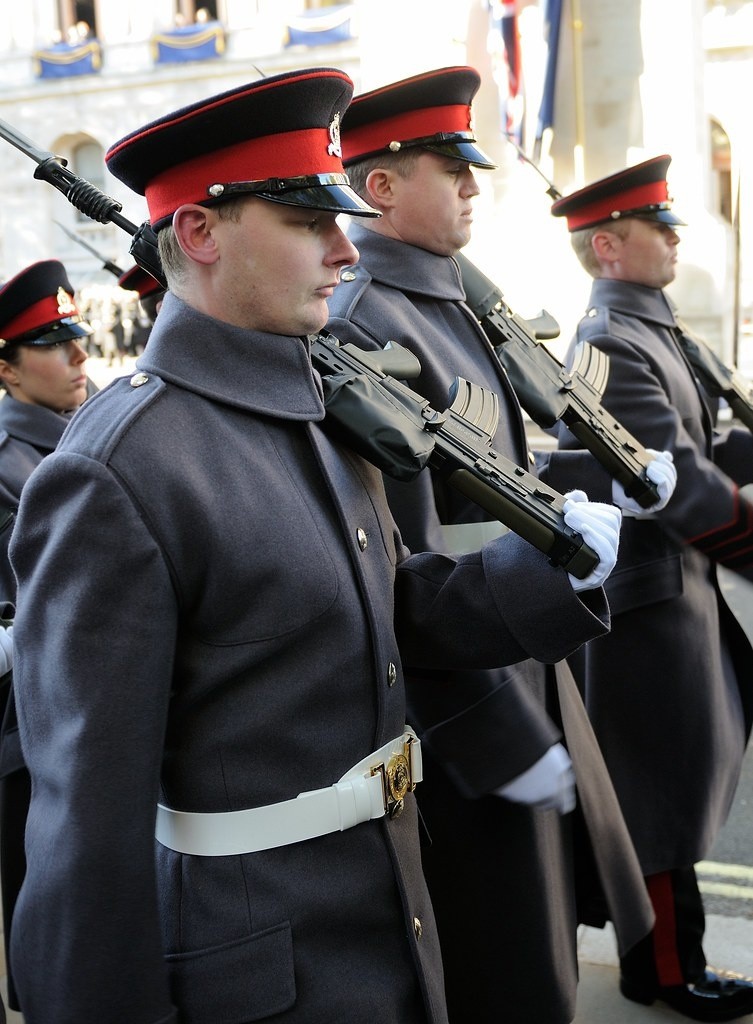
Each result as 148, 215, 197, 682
621, 967, 753, 1021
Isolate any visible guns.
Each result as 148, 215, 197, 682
0, 119, 600, 580
500, 128, 753, 434
454, 251, 660, 509
52, 219, 123, 278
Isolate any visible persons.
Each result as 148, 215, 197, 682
51, 21, 93, 46
174, 9, 215, 27
14, 68, 625, 1024
550, 155, 753, 1024
325, 68, 678, 1024
73, 283, 153, 367
1, 259, 106, 1023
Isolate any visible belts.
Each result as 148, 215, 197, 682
435, 518, 520, 558
152, 723, 422, 856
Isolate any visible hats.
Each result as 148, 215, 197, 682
119, 260, 166, 300
336, 67, 497, 170
0, 259, 92, 349
551, 154, 684, 231
107, 69, 383, 234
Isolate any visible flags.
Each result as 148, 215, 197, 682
479, 0, 563, 153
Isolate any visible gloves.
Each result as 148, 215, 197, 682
546, 489, 622, 594
490, 744, 576, 816
612, 448, 677, 515
0, 625, 15, 678
